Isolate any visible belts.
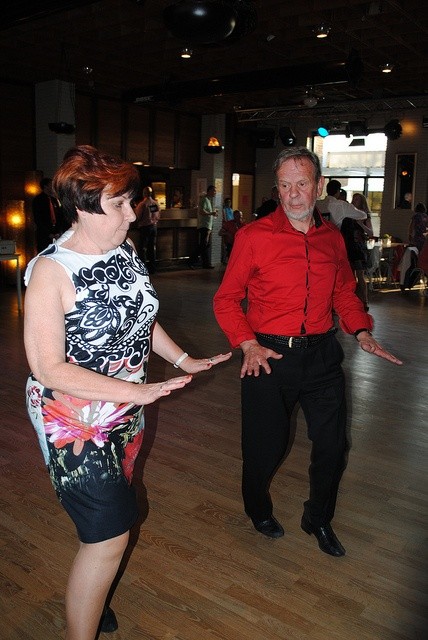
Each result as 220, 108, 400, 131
254, 326, 339, 352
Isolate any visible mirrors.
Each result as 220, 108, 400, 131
392, 152, 417, 212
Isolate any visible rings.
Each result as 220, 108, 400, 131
208, 358, 215, 363
160, 385, 163, 391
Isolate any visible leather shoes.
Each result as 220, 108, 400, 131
251, 513, 284, 537
300, 517, 346, 557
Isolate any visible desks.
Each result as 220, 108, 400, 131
373, 243, 406, 288
0, 253, 21, 310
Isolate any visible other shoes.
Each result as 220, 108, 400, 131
364, 306, 369, 312
202, 265, 215, 269
99, 607, 118, 633
183, 258, 195, 269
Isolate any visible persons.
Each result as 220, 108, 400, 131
197, 185, 218, 269
32, 176, 60, 254
133, 186, 158, 274
316, 181, 374, 312
213, 148, 404, 557
23, 145, 232, 640
218, 184, 279, 266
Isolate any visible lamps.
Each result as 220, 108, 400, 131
315, 22, 331, 38
317, 120, 331, 137
379, 55, 394, 73
305, 83, 322, 108
280, 126, 295, 145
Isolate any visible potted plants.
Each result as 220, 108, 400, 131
382, 232, 392, 247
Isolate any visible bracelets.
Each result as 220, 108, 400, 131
354, 328, 373, 340
174, 353, 188, 368
